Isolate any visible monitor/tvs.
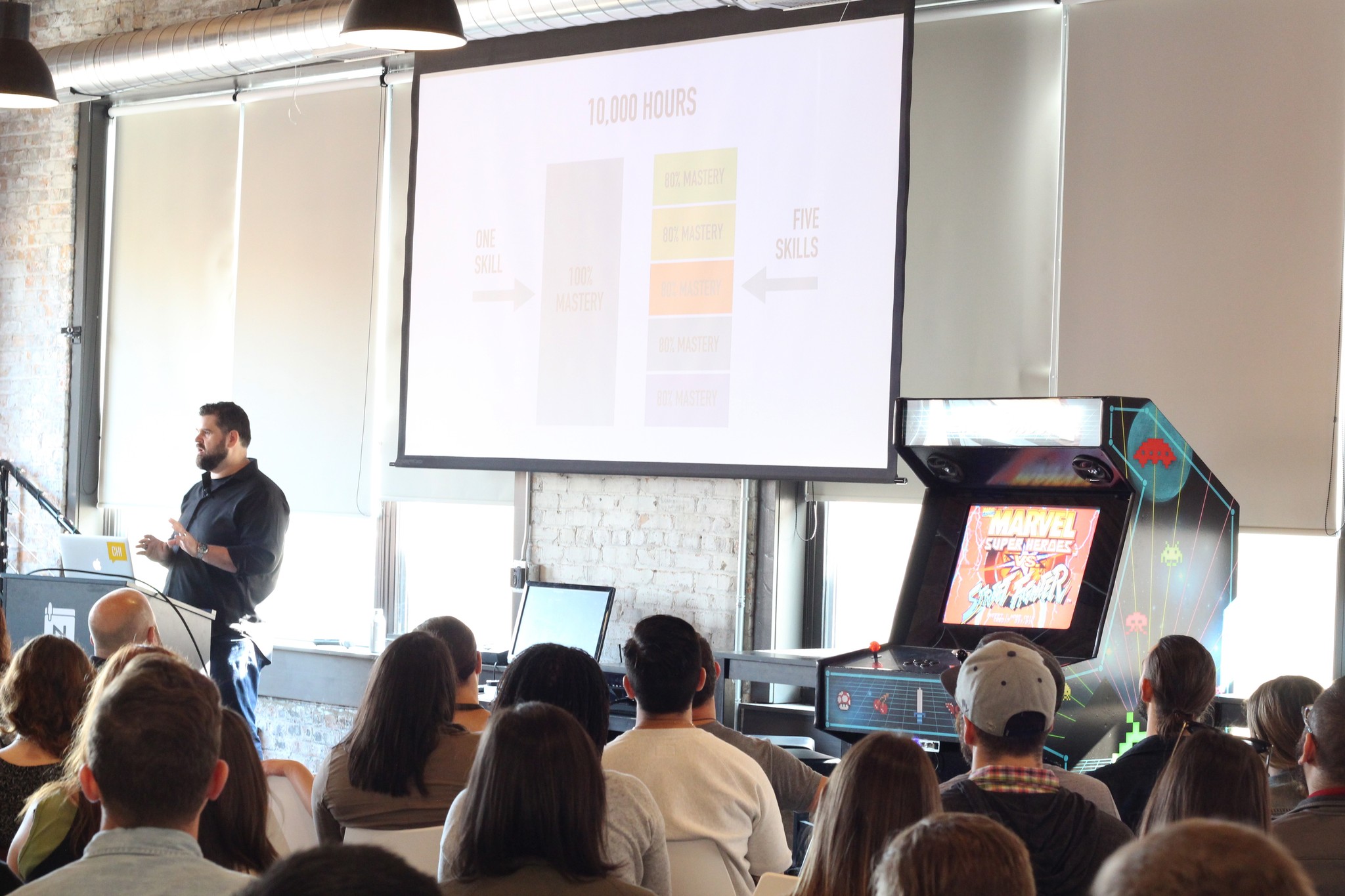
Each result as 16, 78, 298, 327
941, 504, 1101, 629
507, 580, 616, 663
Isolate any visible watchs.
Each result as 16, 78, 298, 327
196, 542, 209, 559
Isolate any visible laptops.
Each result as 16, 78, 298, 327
59, 533, 135, 584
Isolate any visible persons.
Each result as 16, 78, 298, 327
0, 590, 1345, 896
135, 402, 289, 766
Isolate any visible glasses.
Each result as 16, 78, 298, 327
1171, 719, 1275, 775
1301, 702, 1318, 746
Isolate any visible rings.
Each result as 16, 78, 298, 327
183, 532, 187, 536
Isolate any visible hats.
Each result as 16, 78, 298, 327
940, 640, 1056, 741
975, 631, 1065, 712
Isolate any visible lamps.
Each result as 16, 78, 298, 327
340, 0, 467, 50
0, 0, 59, 109
